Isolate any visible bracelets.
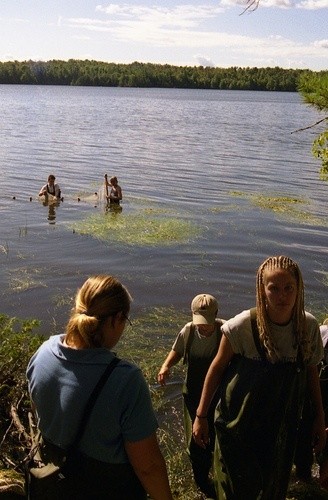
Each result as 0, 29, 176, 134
195, 414, 207, 419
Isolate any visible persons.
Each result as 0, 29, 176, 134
25, 275, 173, 500
39, 175, 61, 201
193, 255, 326, 499
158, 293, 228, 485
104, 176, 122, 206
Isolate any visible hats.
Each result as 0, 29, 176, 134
191, 293, 219, 325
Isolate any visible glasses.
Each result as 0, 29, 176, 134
125, 315, 133, 328
